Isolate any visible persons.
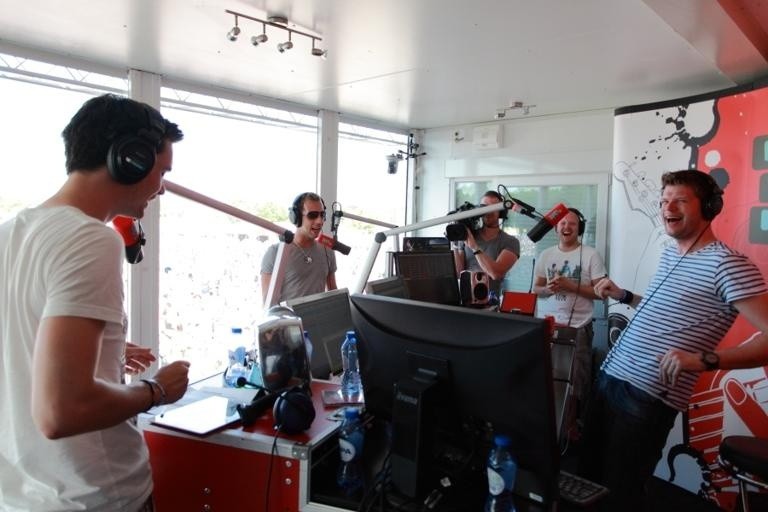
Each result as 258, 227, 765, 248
445, 191, 519, 300
261, 193, 337, 310
534, 208, 606, 349
0, 93, 190, 512
564, 169, 766, 511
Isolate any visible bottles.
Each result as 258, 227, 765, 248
303, 331, 313, 365
339, 408, 365, 490
486, 435, 516, 508
342, 332, 360, 395
227, 328, 245, 386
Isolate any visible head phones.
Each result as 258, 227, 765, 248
702, 169, 724, 221
235, 381, 317, 436
288, 192, 327, 228
106, 101, 167, 186
555, 207, 586, 236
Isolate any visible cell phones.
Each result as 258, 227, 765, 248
322, 389, 344, 407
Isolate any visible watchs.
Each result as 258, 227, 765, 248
473, 248, 484, 256
700, 349, 720, 370
149, 379, 166, 406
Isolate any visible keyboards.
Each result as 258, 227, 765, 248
561, 470, 610, 506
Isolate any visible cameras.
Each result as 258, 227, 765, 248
445, 200, 488, 242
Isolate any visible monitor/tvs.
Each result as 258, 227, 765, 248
279, 288, 359, 384
369, 275, 409, 300
346, 294, 564, 512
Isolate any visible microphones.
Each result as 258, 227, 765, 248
529, 258, 536, 292
528, 203, 570, 243
238, 376, 292, 404
317, 234, 351, 257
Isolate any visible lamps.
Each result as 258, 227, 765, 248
312, 48, 326, 56
226, 27, 240, 42
278, 42, 293, 53
250, 34, 268, 46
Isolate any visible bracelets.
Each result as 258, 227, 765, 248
620, 287, 633, 305
140, 379, 155, 412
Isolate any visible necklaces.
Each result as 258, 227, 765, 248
294, 241, 318, 265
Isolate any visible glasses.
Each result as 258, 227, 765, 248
300, 209, 329, 221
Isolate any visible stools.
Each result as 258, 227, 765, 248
717, 435, 768, 512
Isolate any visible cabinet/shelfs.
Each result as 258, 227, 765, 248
137, 374, 364, 512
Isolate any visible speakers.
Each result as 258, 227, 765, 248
460, 269, 491, 308
